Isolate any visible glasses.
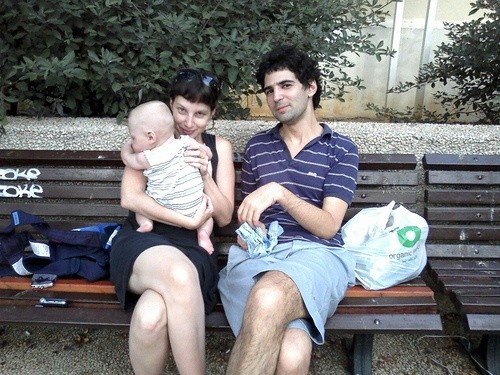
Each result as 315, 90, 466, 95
174, 68, 222, 96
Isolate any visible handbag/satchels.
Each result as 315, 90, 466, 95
341, 199, 430, 290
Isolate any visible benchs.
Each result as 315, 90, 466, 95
0, 148, 500, 375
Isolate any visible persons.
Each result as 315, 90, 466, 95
108, 68, 235, 375
218, 44, 360, 375
120, 100, 214, 255
